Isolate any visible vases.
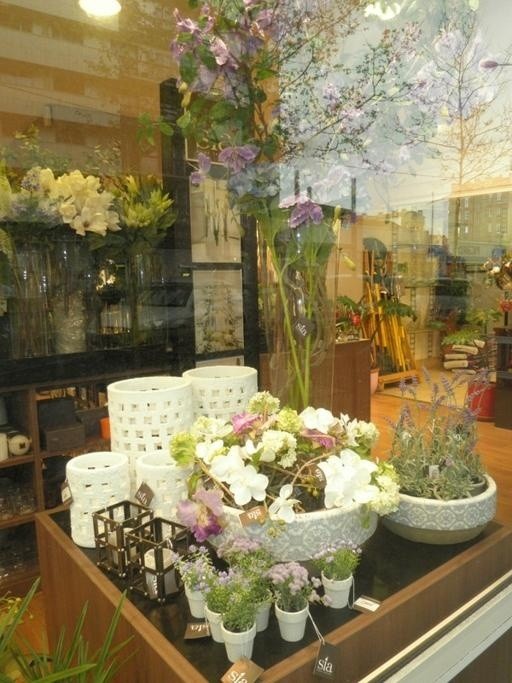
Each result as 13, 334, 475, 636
370, 368, 380, 396
0, 223, 174, 358
203, 597, 235, 643
275, 597, 309, 642
221, 614, 256, 664
380, 460, 498, 545
197, 494, 376, 580
493, 327, 512, 369
240, 584, 272, 632
321, 570, 353, 608
182, 582, 211, 619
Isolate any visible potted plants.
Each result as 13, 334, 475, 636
429, 308, 508, 423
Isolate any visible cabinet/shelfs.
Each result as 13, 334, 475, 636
257, 337, 371, 425
34, 504, 512, 683
1, 354, 181, 609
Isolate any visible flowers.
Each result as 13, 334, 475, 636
482, 256, 512, 330
216, 536, 274, 595
207, 571, 238, 612
333, 297, 416, 370
310, 539, 362, 581
262, 562, 311, 612
386, 363, 500, 499
172, 0, 425, 407
220, 589, 259, 631
171, 389, 401, 543
170, 544, 220, 591
